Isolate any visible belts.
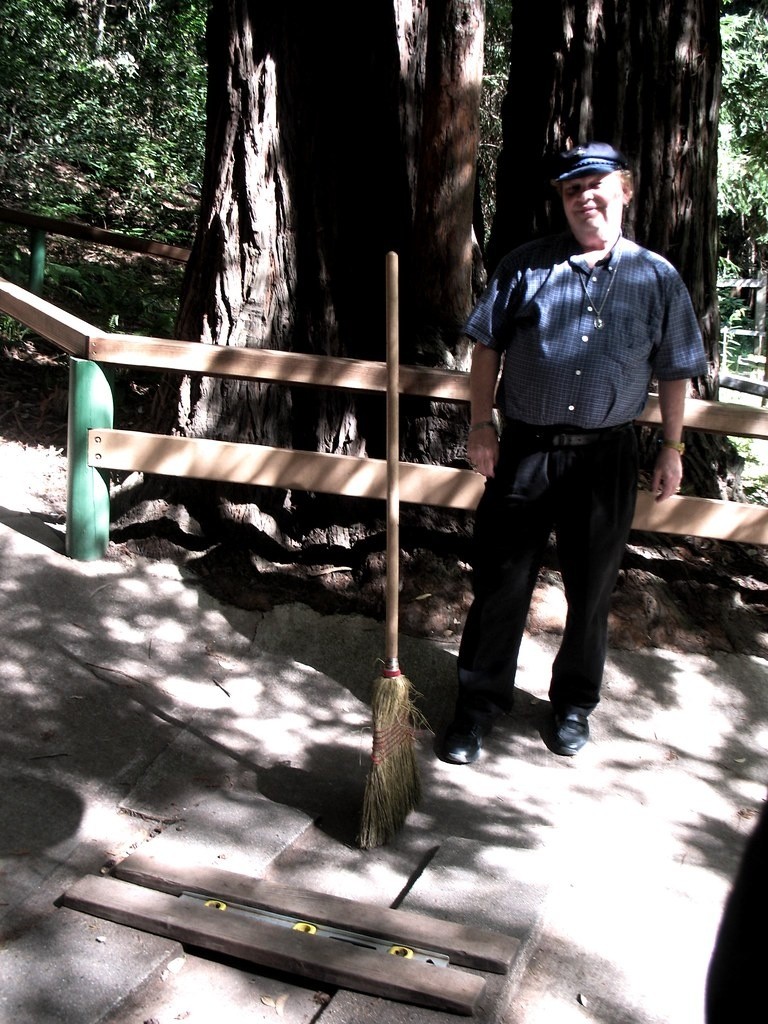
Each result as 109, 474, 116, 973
502, 423, 599, 447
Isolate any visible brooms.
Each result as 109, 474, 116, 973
353, 253, 435, 850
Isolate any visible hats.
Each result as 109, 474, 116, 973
547, 141, 627, 184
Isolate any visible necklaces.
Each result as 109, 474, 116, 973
573, 238, 624, 330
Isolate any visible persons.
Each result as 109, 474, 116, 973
440, 141, 709, 765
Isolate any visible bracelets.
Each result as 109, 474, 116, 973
470, 422, 497, 430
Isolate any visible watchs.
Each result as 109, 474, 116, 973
663, 441, 685, 456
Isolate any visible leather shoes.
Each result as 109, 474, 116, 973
443, 714, 493, 764
551, 708, 589, 755
